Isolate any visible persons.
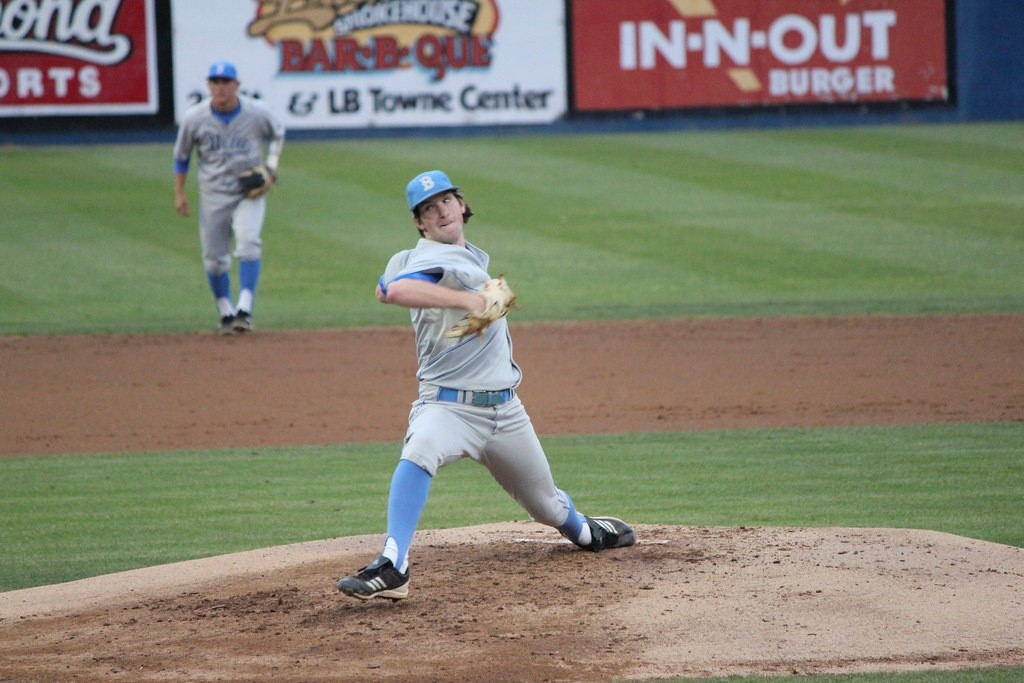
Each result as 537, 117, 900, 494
336, 169, 636, 601
173, 61, 287, 335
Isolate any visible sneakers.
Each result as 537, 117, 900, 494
218, 311, 252, 337
335, 555, 410, 603
570, 510, 635, 549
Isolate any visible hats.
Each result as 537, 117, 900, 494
405, 170, 459, 212
209, 62, 238, 81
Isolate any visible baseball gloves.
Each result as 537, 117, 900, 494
446, 274, 521, 344
236, 164, 276, 197
